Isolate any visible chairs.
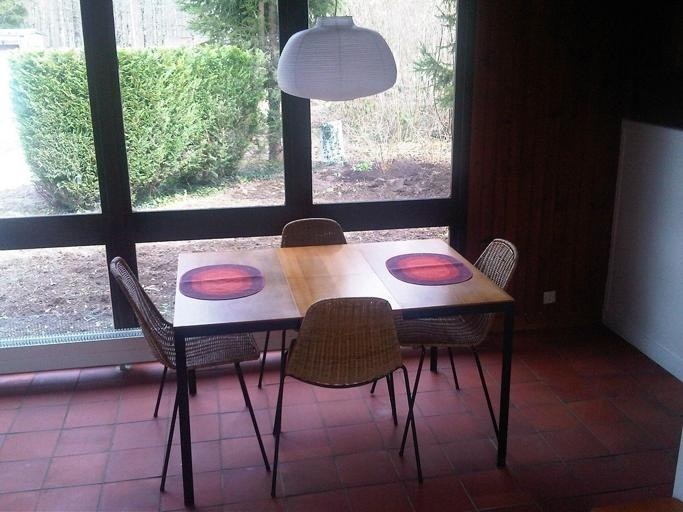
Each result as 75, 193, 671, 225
271, 296, 424, 498
371, 237, 519, 485
110, 255, 273, 492
257, 216, 400, 436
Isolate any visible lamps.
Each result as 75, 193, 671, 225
276, 0, 398, 102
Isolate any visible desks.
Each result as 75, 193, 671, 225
172, 236, 516, 507
